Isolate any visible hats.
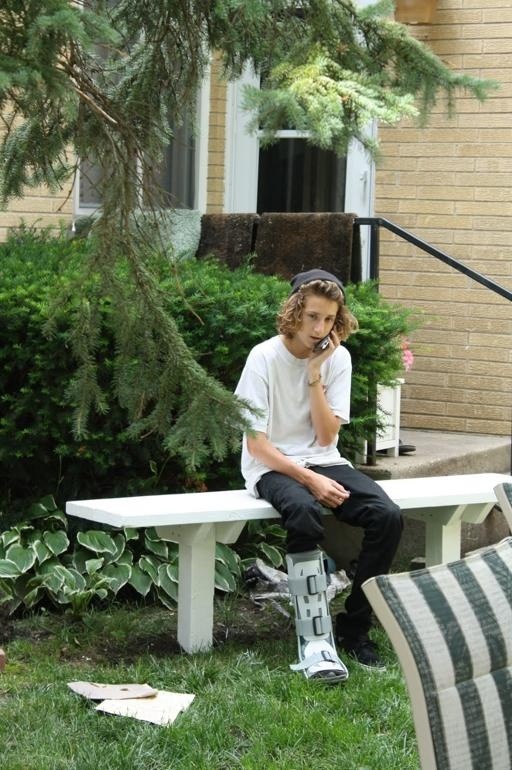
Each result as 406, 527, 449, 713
289, 269, 347, 305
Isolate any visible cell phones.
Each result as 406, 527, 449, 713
312, 335, 330, 354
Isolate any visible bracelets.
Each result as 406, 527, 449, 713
307, 372, 323, 387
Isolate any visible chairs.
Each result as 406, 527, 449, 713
362, 534, 512, 770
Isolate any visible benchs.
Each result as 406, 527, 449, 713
65, 470, 512, 656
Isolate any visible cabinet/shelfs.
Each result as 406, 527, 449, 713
344, 377, 406, 463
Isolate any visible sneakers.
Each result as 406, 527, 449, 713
336, 633, 386, 672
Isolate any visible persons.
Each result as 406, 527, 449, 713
225, 266, 404, 684
